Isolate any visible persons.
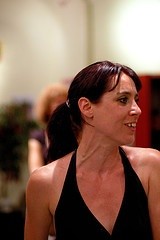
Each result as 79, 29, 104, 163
27, 84, 69, 175
22, 59, 160, 240
